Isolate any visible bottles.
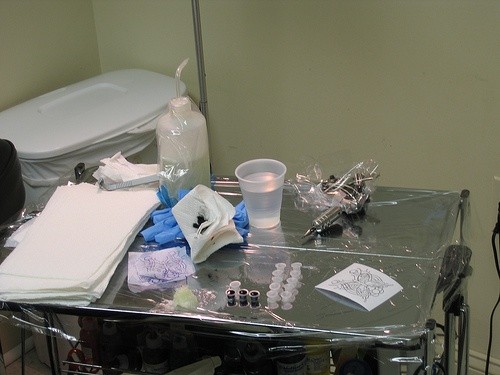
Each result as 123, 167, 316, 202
158, 98, 210, 204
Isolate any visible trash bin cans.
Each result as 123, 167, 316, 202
0, 68, 206, 375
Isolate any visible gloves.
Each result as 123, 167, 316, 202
141, 188, 250, 246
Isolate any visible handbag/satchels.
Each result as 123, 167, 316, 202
0, 139, 25, 226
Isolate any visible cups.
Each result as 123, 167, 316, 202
235, 158, 287, 229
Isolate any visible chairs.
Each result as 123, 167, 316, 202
0, 138, 61, 375
